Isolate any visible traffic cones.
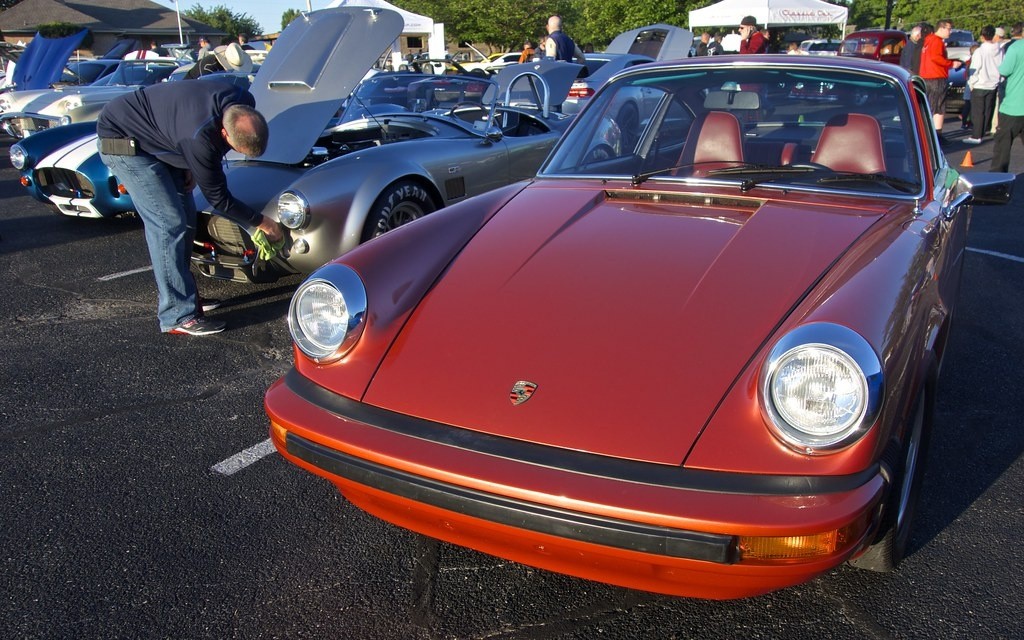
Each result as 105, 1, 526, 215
959, 150, 974, 167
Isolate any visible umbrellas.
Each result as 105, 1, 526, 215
782, 32, 810, 42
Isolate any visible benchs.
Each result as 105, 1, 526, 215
743, 141, 813, 166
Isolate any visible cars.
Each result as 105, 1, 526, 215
0, 28, 272, 220
798, 30, 978, 91
262, 54, 1015, 601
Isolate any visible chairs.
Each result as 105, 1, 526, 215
667, 111, 746, 179
811, 112, 885, 175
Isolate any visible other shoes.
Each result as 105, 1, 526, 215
961, 123, 971, 128
962, 137, 982, 144
938, 135, 952, 145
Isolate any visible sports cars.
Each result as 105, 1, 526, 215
184, 6, 720, 284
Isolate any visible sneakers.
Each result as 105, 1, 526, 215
166, 313, 226, 335
202, 298, 220, 311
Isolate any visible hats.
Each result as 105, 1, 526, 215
741, 16, 757, 26
995, 27, 1005, 37
213, 42, 253, 74
198, 37, 208, 44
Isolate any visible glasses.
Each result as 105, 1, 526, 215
739, 26, 746, 29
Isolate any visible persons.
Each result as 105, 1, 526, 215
738, 15, 779, 53
954, 23, 1004, 142
912, 24, 948, 76
445, 46, 449, 55
417, 49, 425, 60
196, 35, 215, 60
705, 31, 724, 56
990, 27, 1024, 173
405, 49, 413, 62
97, 80, 283, 335
150, 41, 171, 57
920, 19, 964, 146
788, 40, 809, 55
899, 25, 924, 75
544, 15, 585, 113
238, 33, 256, 50
412, 59, 424, 74
399, 64, 410, 72
533, 35, 547, 60
694, 32, 708, 56
385, 60, 394, 72
518, 40, 535, 65
997, 22, 1024, 106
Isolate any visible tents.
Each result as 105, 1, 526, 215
325, 0, 434, 48
688, 0, 849, 53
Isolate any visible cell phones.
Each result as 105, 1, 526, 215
101, 138, 136, 155
750, 28, 754, 33
